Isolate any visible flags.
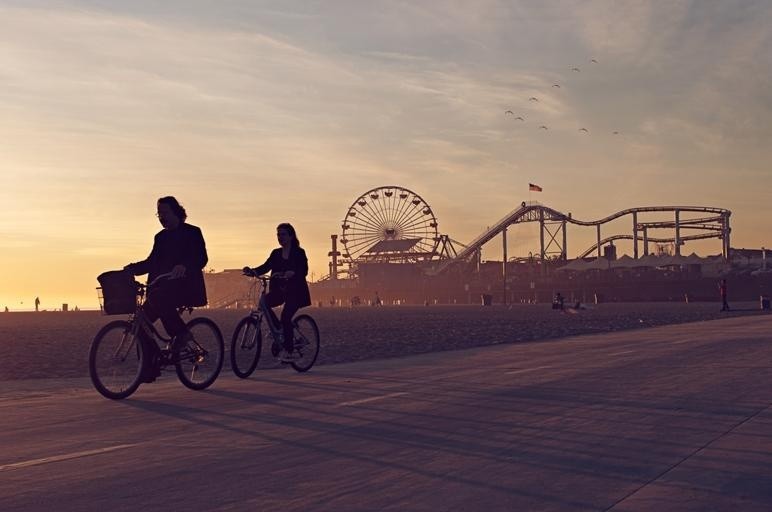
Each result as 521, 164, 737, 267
528, 182, 543, 193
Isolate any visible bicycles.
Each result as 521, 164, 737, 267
230, 267, 320, 380
89, 266, 225, 400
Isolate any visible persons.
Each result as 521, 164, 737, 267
717, 278, 730, 312
684, 292, 689, 304
678, 286, 685, 302
33, 296, 44, 312
553, 291, 562, 309
242, 222, 314, 362
594, 289, 599, 305
122, 195, 210, 383
326, 288, 538, 308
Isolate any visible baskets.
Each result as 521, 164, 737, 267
97, 285, 135, 314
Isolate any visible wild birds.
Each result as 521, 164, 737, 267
572, 68, 581, 72
515, 116, 524, 121
592, 58, 599, 64
580, 128, 589, 132
552, 84, 561, 88
613, 131, 623, 136
539, 126, 548, 130
505, 110, 513, 115
528, 97, 538, 101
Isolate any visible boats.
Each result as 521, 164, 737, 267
552, 298, 564, 310
561, 304, 587, 318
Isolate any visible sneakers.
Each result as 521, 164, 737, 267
173, 330, 192, 347
283, 352, 295, 361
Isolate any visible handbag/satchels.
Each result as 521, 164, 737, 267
97, 271, 135, 313
